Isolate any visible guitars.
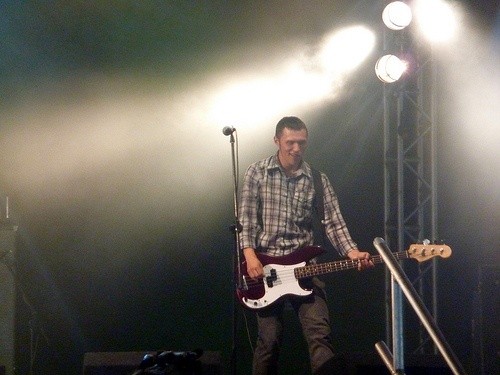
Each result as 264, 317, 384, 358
236, 239, 452, 309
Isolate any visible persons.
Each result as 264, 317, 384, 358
240, 117, 375, 375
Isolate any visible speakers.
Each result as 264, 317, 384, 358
83, 351, 222, 375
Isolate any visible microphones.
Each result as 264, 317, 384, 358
223, 126, 236, 136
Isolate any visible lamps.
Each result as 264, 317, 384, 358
374, 54, 410, 84
